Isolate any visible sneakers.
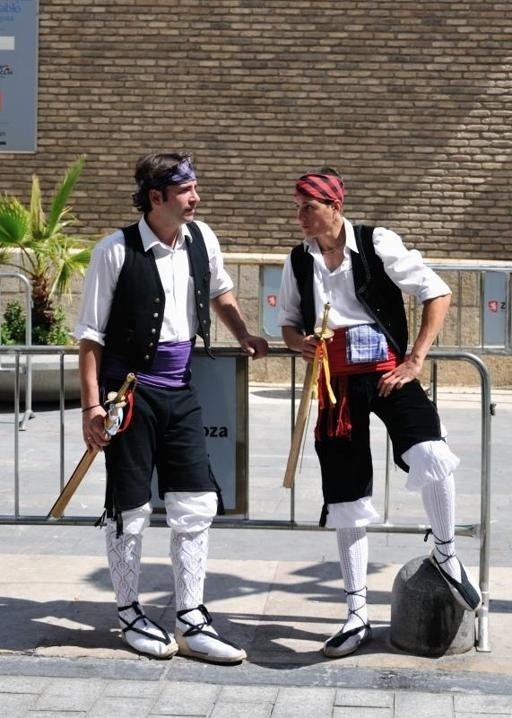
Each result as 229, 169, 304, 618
430, 546, 482, 612
175, 627, 246, 662
118, 614, 179, 659
324, 620, 372, 656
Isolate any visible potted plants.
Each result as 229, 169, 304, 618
0, 155, 106, 402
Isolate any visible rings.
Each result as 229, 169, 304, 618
86, 435, 93, 441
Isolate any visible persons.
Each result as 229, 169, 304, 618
74, 153, 269, 662
279, 167, 481, 658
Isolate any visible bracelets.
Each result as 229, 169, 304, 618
79, 402, 101, 413
